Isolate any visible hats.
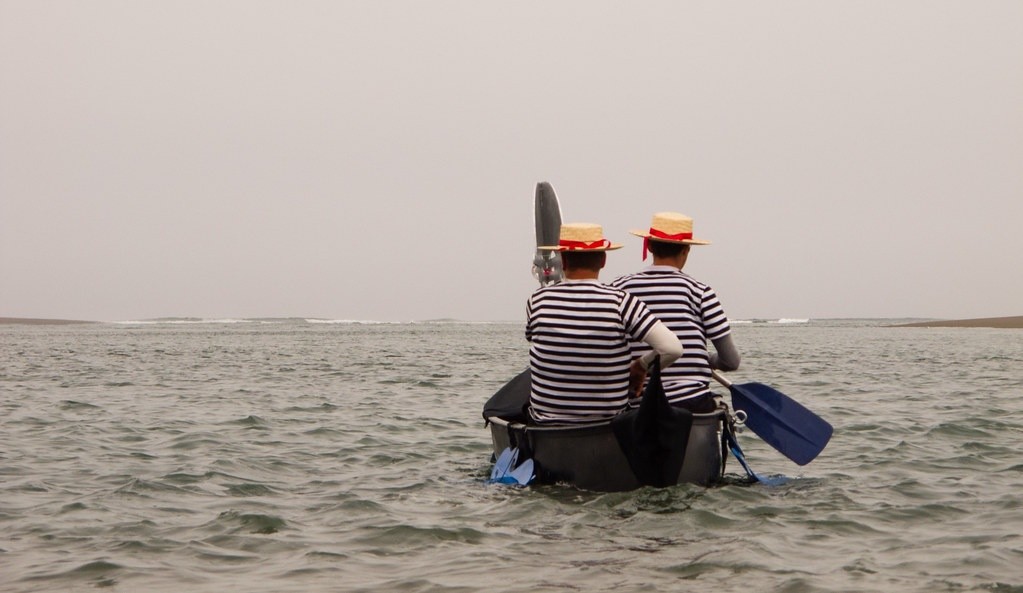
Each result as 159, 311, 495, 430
537, 225, 625, 252
629, 212, 711, 245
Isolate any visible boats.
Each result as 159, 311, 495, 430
481, 365, 734, 486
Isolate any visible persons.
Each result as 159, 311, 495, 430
605, 212, 740, 416
525, 224, 687, 426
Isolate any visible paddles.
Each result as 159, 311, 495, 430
712, 368, 834, 465
